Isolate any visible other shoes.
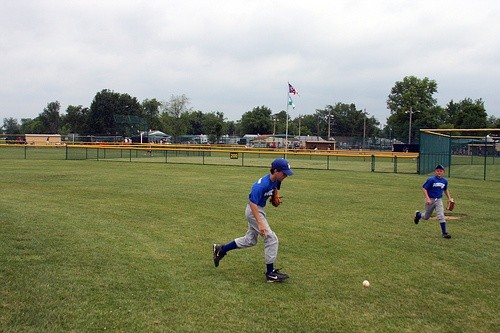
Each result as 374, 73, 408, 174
414, 211, 421, 224
441, 233, 451, 238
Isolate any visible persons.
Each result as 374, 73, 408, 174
5, 136, 409, 157
414, 164, 453, 238
213, 159, 294, 282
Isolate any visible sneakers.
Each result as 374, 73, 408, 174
266, 269, 289, 282
213, 244, 226, 267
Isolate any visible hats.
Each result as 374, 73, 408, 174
436, 165, 444, 170
271, 158, 294, 175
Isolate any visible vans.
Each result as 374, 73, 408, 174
6, 133, 26, 144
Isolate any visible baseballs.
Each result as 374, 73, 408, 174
362, 279, 370, 288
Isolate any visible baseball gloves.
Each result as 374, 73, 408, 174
271, 186, 281, 207
446, 200, 455, 211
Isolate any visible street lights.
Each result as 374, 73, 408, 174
271, 114, 279, 137
325, 109, 335, 139
404, 107, 420, 143
360, 107, 370, 147
296, 113, 304, 138
139, 129, 152, 144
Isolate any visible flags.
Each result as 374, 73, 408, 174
288, 83, 298, 95
288, 96, 295, 109
